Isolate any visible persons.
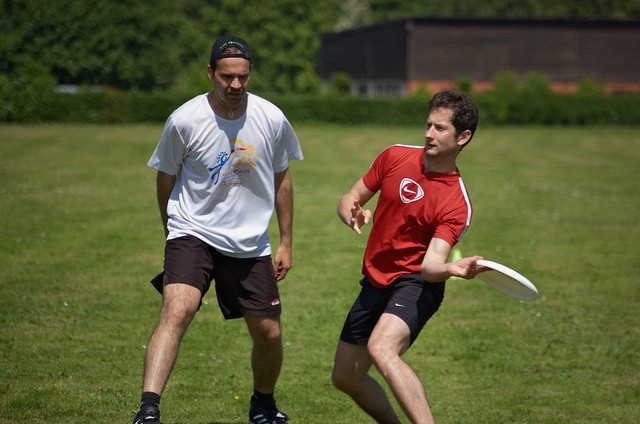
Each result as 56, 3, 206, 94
332, 89, 491, 424
131, 37, 304, 424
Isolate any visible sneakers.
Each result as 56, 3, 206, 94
132, 405, 163, 424
249, 395, 289, 424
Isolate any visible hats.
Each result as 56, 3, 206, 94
210, 36, 252, 64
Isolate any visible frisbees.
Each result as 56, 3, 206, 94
475, 260, 538, 302
